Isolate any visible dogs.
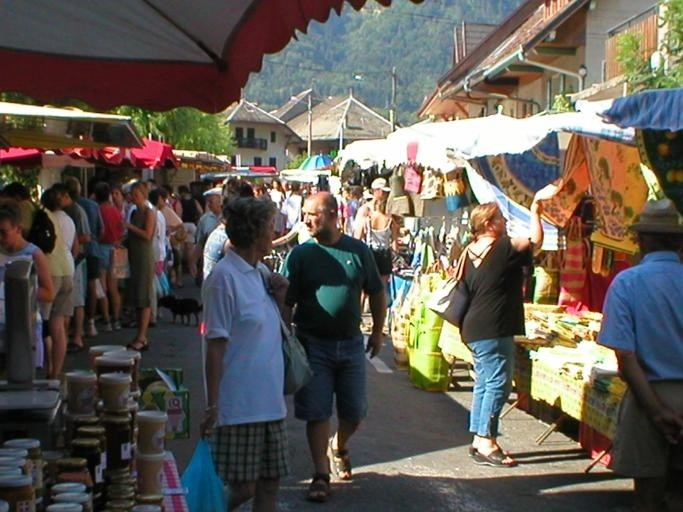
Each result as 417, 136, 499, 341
159, 294, 203, 327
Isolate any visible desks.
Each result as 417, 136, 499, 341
438, 309, 633, 474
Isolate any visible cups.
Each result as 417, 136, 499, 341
132, 409, 168, 496
64, 344, 141, 414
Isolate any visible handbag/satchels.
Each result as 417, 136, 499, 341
443, 170, 470, 210
532, 252, 558, 304
559, 214, 592, 309
390, 309, 410, 370
419, 166, 443, 199
282, 321, 317, 396
25, 199, 57, 254
32, 287, 44, 368
426, 242, 477, 328
388, 165, 424, 232
110, 244, 129, 278
369, 245, 394, 276
407, 349, 447, 391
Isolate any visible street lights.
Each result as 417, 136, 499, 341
290, 96, 312, 159
352, 65, 398, 133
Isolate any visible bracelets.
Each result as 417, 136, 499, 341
203, 402, 216, 411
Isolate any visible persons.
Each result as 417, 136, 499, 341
278, 190, 390, 505
595, 197, 683, 511
192, 196, 290, 510
452, 200, 545, 468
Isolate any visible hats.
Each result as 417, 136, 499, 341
202, 186, 224, 197
370, 176, 392, 193
628, 198, 682, 234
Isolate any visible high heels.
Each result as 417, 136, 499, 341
469, 445, 516, 467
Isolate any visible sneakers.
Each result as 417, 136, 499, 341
85, 317, 157, 337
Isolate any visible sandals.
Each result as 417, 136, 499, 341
66, 341, 87, 355
127, 338, 151, 353
305, 471, 332, 502
327, 437, 352, 481
